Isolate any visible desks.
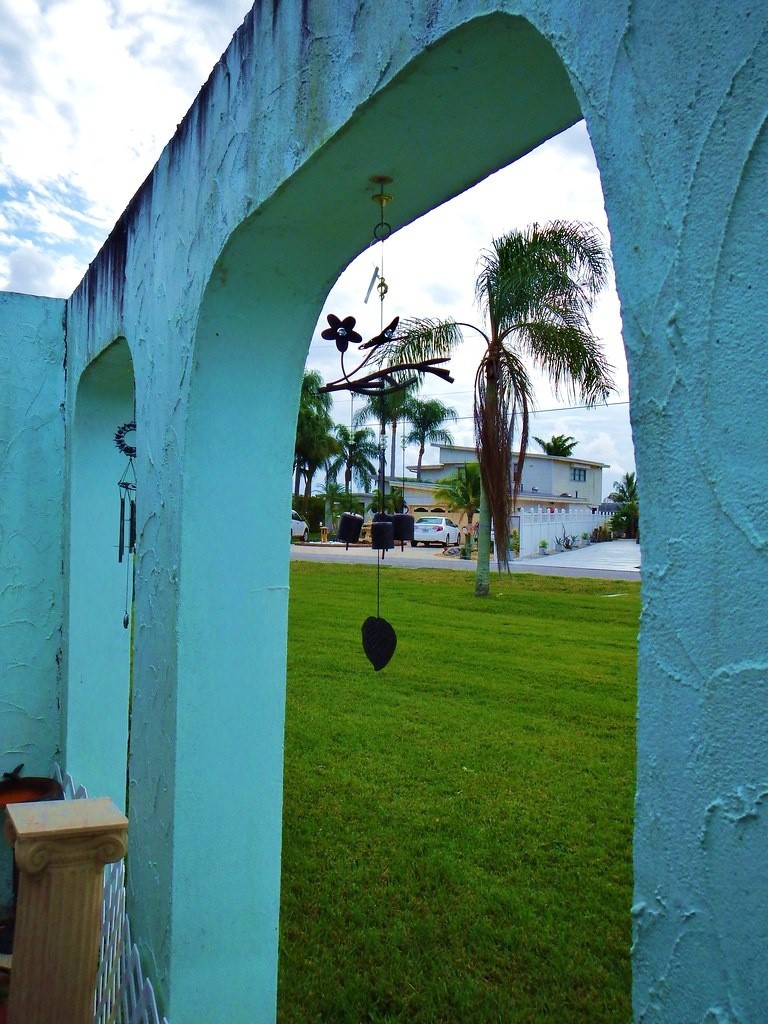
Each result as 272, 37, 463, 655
4, 797, 129, 1024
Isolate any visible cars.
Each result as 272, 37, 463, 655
411, 516, 461, 548
290, 509, 310, 544
